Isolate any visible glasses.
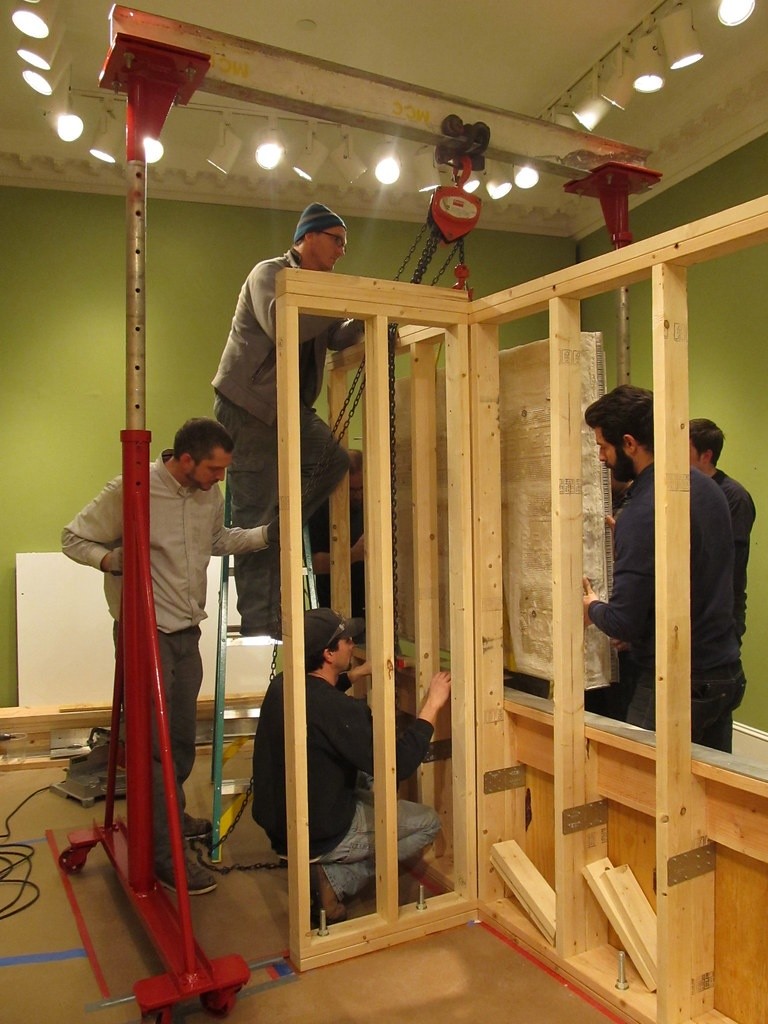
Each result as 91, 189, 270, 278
315, 230, 347, 253
327, 618, 347, 646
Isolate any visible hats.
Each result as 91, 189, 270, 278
294, 202, 347, 243
304, 607, 365, 649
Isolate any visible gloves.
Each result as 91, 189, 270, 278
263, 518, 283, 543
103, 548, 126, 576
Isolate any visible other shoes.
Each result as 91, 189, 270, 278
309, 863, 347, 925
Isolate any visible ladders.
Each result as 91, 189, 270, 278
210, 470, 319, 863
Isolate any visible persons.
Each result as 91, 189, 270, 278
211, 201, 353, 638
62, 417, 281, 896
253, 612, 453, 927
584, 387, 747, 751
689, 419, 757, 653
307, 450, 367, 646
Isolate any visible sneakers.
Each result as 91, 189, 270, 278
181, 811, 212, 840
155, 857, 217, 895
